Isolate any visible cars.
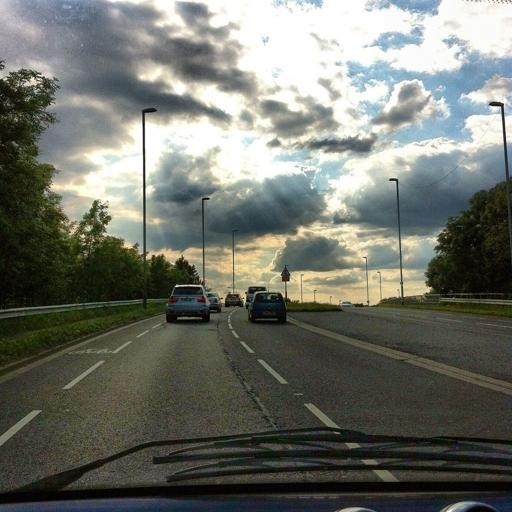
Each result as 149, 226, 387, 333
224, 295, 244, 307
245, 286, 286, 323
340, 302, 352, 307
167, 285, 223, 321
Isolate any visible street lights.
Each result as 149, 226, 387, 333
233, 228, 240, 295
203, 197, 210, 285
300, 273, 332, 304
362, 178, 403, 307
489, 102, 512, 266
142, 108, 156, 310
